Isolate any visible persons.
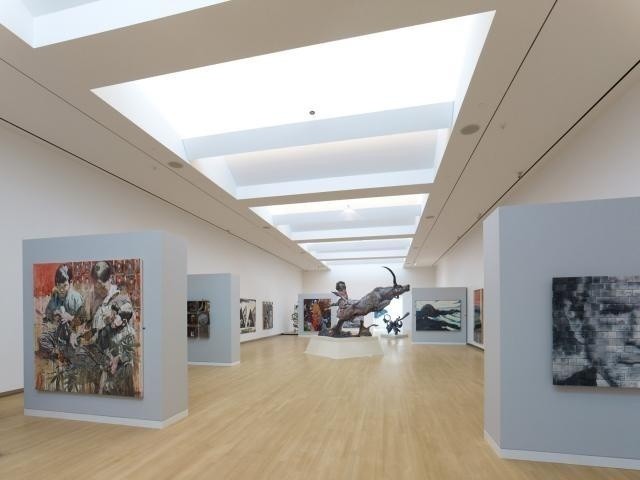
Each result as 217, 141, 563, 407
37, 264, 86, 358
88, 300, 137, 396
70, 262, 136, 354
